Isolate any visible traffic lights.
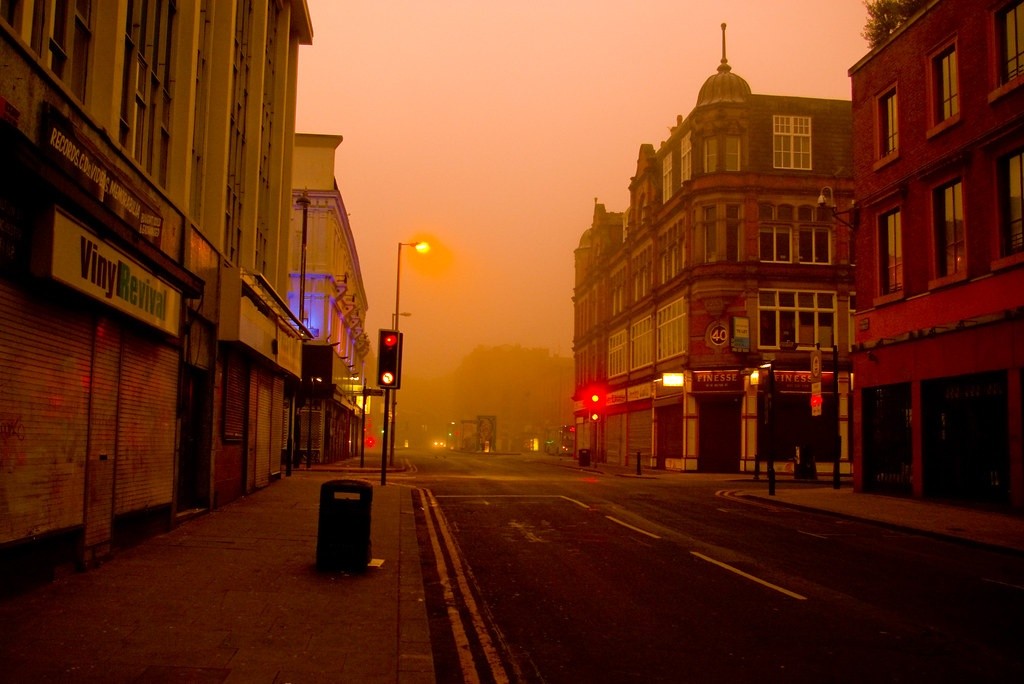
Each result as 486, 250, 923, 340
590, 390, 602, 424
377, 329, 403, 391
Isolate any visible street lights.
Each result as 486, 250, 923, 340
750, 358, 777, 496
380, 241, 430, 484
389, 312, 413, 464
284, 186, 311, 478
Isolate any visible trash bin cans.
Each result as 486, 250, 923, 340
579, 449, 590, 467
793, 441, 817, 480
316, 480, 372, 573
